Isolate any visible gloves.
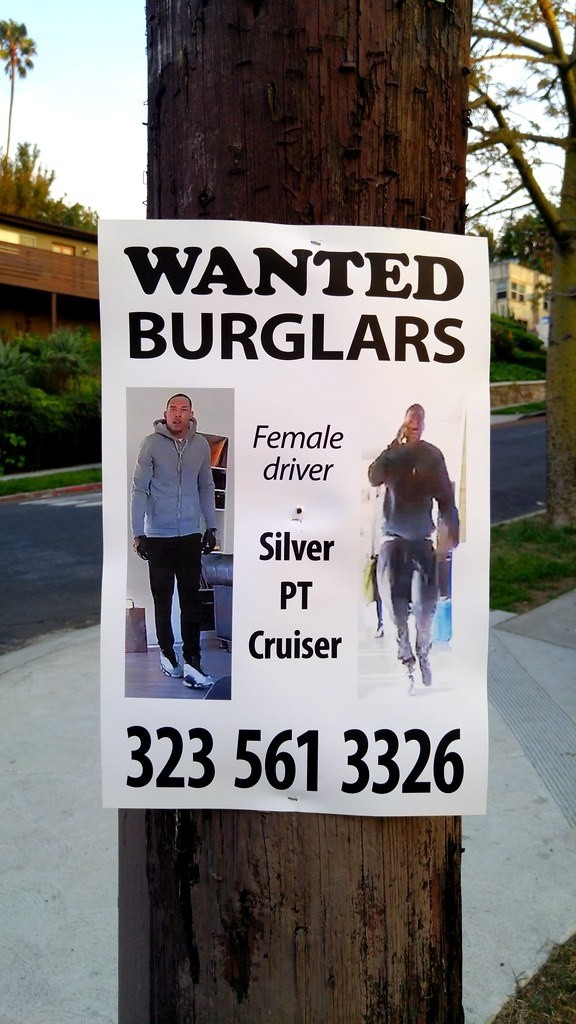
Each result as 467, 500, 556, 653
133, 535, 150, 560
201, 528, 218, 555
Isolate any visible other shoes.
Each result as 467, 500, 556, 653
374, 623, 383, 638
417, 655, 430, 685
407, 661, 417, 695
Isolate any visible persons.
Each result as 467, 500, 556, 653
131, 392, 216, 689
367, 400, 460, 691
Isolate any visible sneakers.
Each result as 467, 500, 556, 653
160, 651, 184, 679
185, 663, 214, 688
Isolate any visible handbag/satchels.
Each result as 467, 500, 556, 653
126, 598, 148, 653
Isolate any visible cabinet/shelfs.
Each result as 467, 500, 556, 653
173, 432, 230, 643
